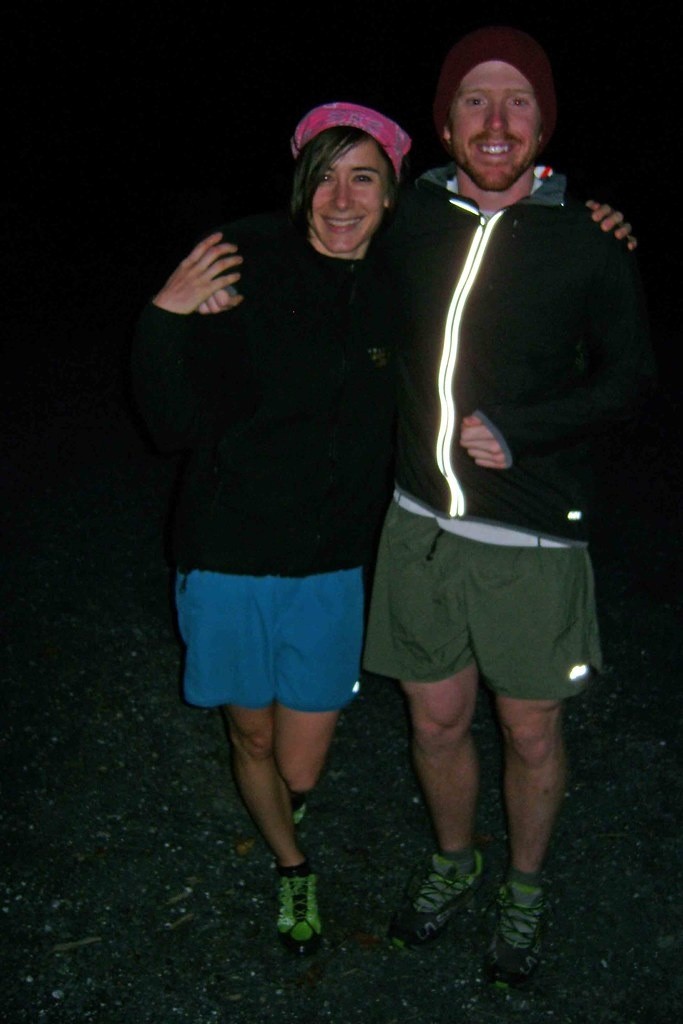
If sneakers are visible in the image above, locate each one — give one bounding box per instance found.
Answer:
[275,850,321,954]
[289,789,307,827]
[481,862,553,993]
[386,849,484,953]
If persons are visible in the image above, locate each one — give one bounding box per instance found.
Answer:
[191,26,634,990]
[133,101,638,951]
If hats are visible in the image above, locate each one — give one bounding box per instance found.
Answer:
[433,26,556,154]
[291,102,412,183]
